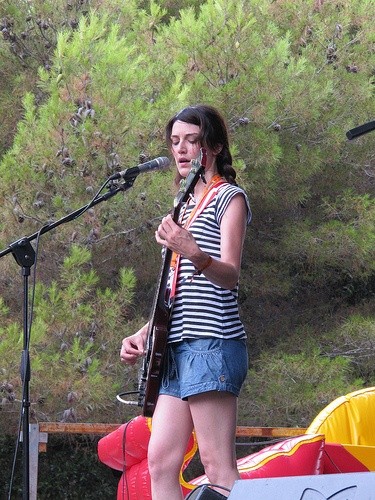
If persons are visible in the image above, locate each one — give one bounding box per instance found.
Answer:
[116,104,253,500]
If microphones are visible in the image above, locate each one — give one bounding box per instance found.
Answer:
[109,157,170,180]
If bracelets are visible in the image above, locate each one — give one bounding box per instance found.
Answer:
[194,252,212,276]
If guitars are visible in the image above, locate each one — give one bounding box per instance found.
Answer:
[138,148,207,417]
